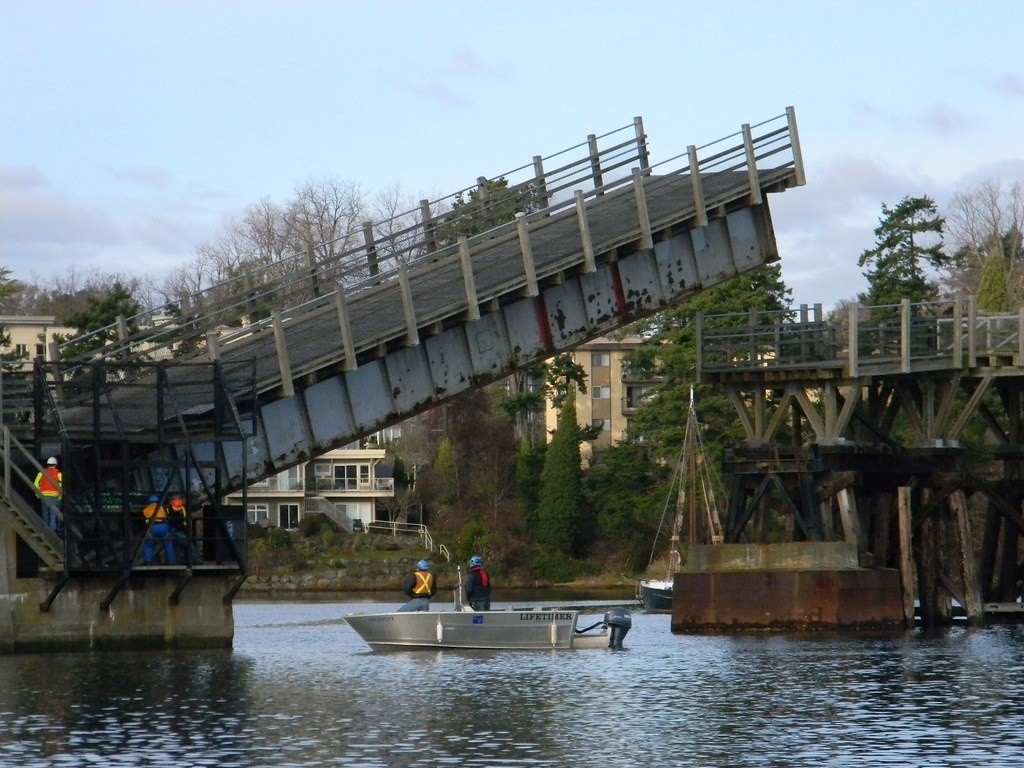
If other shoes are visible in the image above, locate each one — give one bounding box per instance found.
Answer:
[194,560,205,565]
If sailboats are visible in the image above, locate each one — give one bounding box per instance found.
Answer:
[638,386,725,613]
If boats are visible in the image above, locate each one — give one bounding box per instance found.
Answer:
[341,607,631,650]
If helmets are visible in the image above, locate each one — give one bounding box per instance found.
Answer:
[172,500,183,510]
[46,457,57,465]
[469,557,482,565]
[148,496,159,503]
[417,560,429,569]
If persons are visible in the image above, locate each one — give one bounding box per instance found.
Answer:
[143,492,178,566]
[167,499,204,566]
[34,457,62,532]
[395,560,437,613]
[464,556,490,612]
